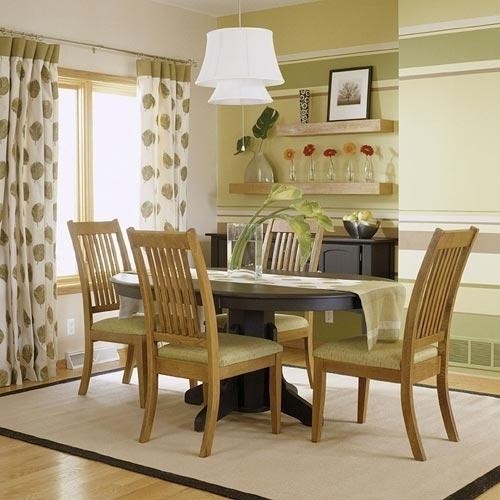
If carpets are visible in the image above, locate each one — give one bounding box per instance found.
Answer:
[0,362,499,500]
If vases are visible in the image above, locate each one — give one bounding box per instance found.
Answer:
[287,155,373,183]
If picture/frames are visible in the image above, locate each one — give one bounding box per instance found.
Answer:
[326,66,372,121]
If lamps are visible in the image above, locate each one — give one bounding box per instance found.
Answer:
[195,0,286,151]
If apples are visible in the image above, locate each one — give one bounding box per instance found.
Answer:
[342,210,378,225]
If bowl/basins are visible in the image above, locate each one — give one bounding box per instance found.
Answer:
[343,219,381,239]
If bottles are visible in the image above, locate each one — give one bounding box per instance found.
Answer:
[244,151,375,183]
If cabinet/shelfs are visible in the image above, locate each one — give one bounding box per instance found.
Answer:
[228,117,396,196]
[205,232,398,279]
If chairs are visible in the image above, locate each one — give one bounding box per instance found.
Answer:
[204,218,324,388]
[66,218,196,408]
[125,226,284,458]
[312,224,481,460]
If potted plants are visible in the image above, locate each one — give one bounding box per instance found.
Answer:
[233,107,279,183]
[225,183,334,278]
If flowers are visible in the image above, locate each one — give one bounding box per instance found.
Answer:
[282,143,375,180]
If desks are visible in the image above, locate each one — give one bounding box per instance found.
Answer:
[111,267,400,432]
